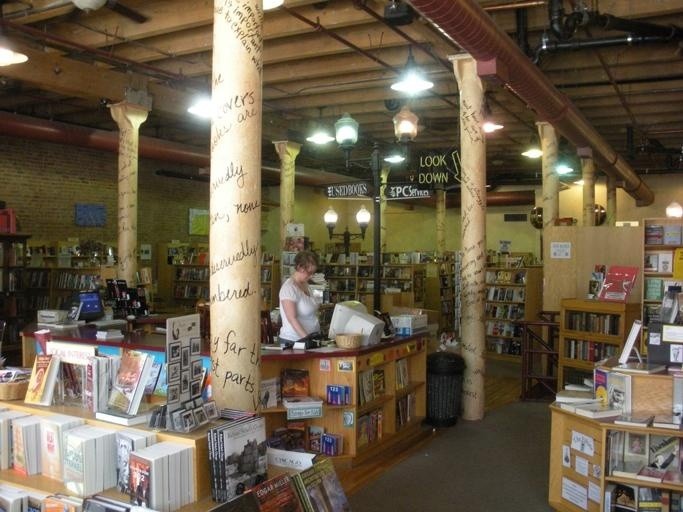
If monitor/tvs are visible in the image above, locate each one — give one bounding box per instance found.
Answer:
[328,299,386,346]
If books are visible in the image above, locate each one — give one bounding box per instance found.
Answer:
[2,223,527,511]
[552,222,682,512]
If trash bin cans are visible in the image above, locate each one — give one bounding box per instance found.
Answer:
[426,352,466,428]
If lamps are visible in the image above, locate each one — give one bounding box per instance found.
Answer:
[305,45,543,162]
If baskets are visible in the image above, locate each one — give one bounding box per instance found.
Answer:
[331,327,364,349]
